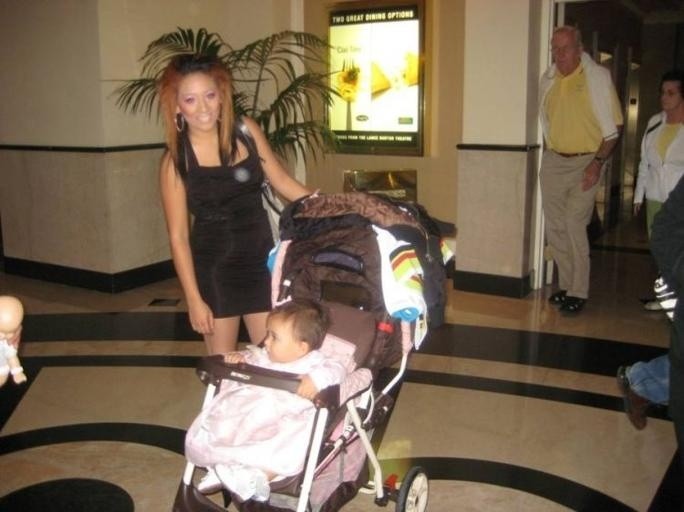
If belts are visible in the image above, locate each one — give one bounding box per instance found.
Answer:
[551,148,594,158]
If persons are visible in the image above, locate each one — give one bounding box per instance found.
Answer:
[158,55,315,356]
[633,69,683,311]
[539,25,624,316]
[617,353,671,430]
[648,174,683,512]
[184,297,346,503]
[7,328,21,349]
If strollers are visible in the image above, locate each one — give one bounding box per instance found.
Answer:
[168,192,446,510]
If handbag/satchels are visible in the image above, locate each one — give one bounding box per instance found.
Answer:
[260,179,285,248]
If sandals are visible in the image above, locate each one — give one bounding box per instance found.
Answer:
[562,297,585,313]
[548,290,567,304]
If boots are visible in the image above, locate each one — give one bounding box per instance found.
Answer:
[637,279,657,301]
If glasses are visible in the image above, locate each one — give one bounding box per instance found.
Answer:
[551,45,581,53]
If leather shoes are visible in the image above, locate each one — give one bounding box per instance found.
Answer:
[617,365,652,431]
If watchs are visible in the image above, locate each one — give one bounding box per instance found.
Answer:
[594,155,605,165]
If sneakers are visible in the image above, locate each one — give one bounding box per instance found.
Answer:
[214,463,257,503]
[643,299,663,311]
[197,469,223,495]
